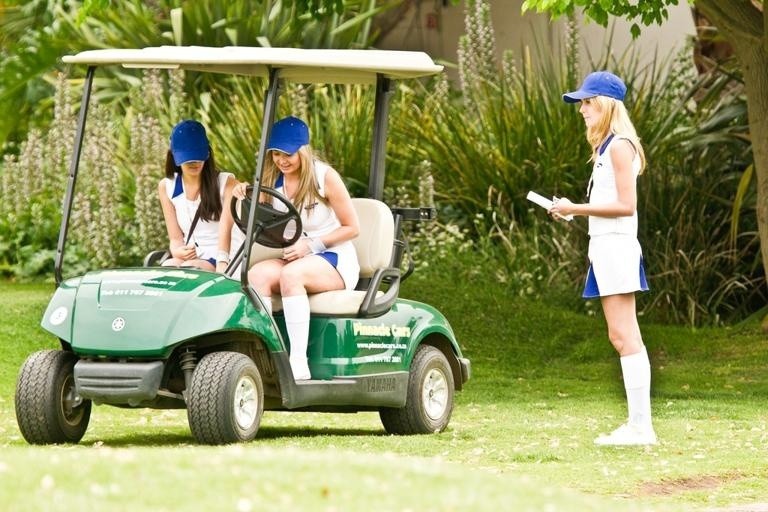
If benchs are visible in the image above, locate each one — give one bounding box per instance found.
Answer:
[143,198,403,319]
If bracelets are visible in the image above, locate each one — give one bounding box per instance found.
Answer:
[306,238,327,254]
[216,251,229,264]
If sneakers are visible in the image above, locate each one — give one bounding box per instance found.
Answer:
[598,425,655,445]
[279,353,311,383]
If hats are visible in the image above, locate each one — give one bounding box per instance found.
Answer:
[266,117,310,155]
[561,70,628,104]
[170,120,211,166]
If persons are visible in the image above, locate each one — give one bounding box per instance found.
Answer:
[224,115,363,377]
[155,118,244,281]
[547,70,660,446]
